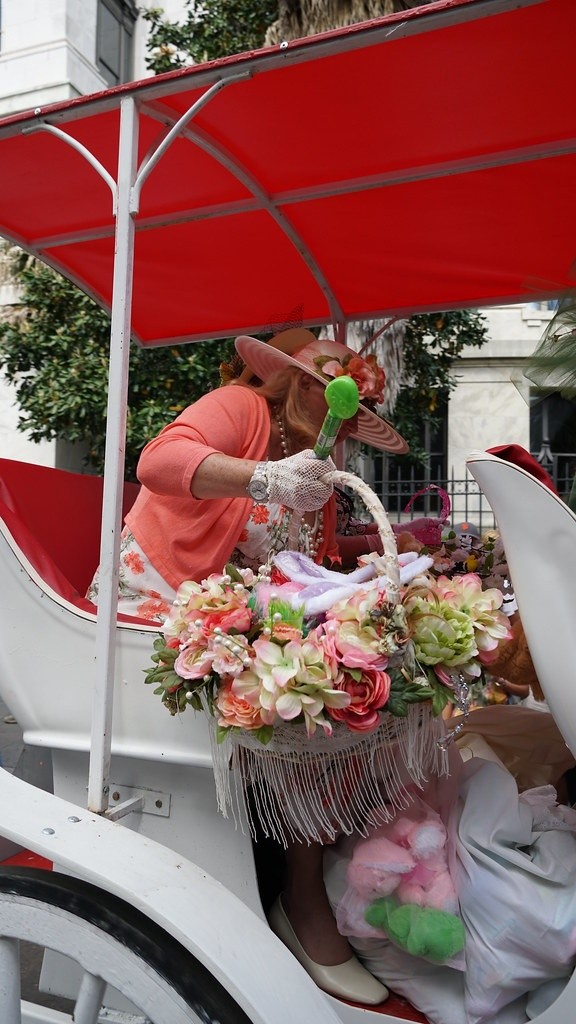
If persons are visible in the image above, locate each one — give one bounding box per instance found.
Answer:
[81,337,452,1010]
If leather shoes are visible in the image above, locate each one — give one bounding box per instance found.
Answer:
[268,891,390,1005]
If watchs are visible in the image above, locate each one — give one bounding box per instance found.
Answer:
[245,461,270,505]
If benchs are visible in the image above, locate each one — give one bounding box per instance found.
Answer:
[0,456,241,770]
[465,444,576,760]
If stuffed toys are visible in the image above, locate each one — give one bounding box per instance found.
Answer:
[336,816,464,963]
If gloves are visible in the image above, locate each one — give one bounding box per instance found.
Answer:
[365,530,425,553]
[392,518,450,545]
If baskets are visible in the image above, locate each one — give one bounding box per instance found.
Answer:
[216,469,435,761]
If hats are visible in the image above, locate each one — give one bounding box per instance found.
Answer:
[235,335,410,455]
[454,522,480,540]
[219,304,317,388]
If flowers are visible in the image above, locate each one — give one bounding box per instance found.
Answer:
[313,351,386,406]
[146,527,515,744]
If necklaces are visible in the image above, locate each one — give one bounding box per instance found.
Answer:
[273,404,323,562]
